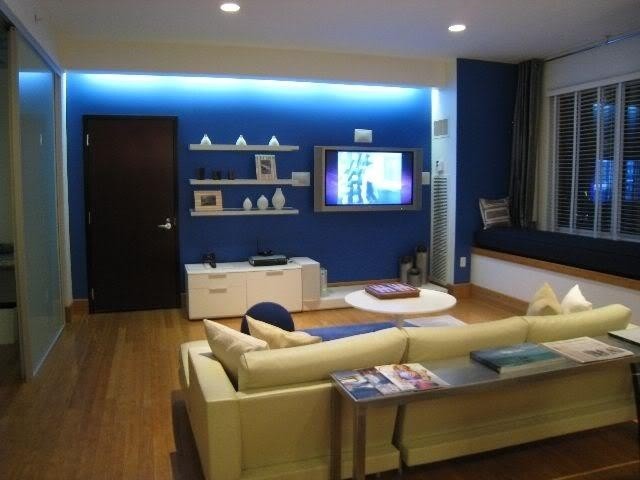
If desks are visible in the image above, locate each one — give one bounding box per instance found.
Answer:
[327,333,640,479]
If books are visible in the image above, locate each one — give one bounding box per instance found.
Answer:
[542,337,633,363]
[469,343,567,376]
[374,363,451,392]
[329,368,382,401]
[611,324,640,344]
[356,368,402,397]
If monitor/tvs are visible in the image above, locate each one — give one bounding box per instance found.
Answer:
[312,144,422,213]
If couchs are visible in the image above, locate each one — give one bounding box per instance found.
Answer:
[179,303,640,479]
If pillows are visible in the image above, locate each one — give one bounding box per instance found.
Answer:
[200,319,271,394]
[526,284,559,317]
[478,196,513,230]
[246,315,322,349]
[560,284,593,315]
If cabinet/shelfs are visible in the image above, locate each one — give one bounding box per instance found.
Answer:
[184,261,303,320]
[188,145,301,217]
